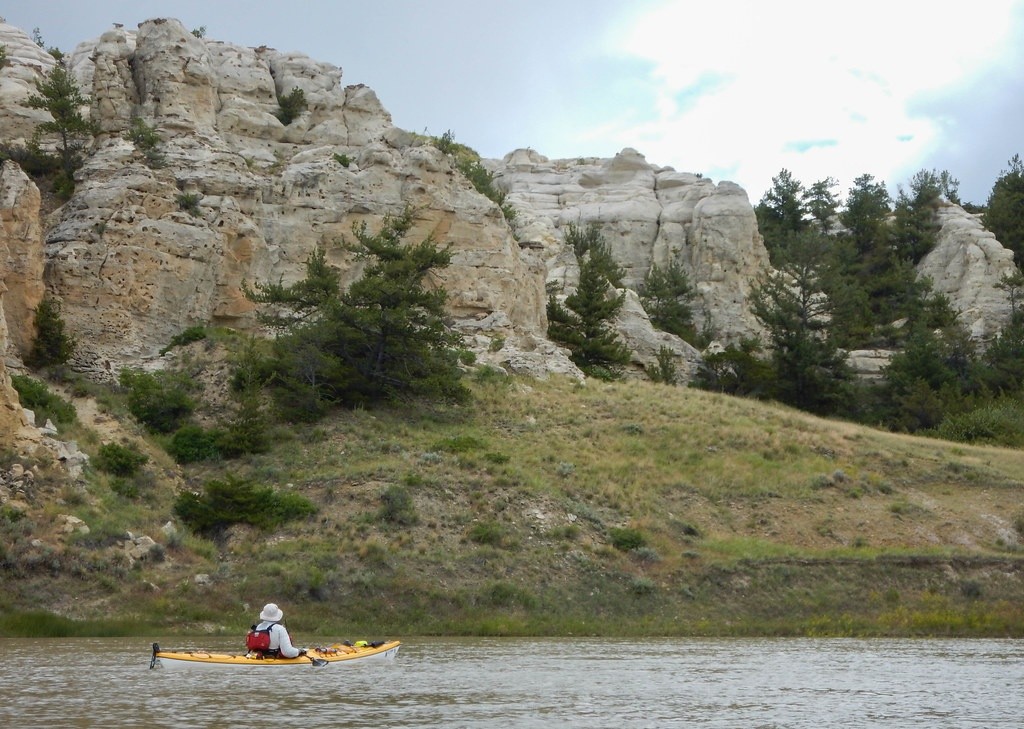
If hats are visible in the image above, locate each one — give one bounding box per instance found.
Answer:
[260,603,284,622]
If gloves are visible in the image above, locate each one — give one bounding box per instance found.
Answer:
[298,649,307,657]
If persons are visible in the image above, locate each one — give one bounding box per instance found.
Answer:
[255,603,309,659]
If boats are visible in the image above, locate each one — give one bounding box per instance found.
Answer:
[149,640,403,669]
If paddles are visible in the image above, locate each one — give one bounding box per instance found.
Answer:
[251,624,330,668]
